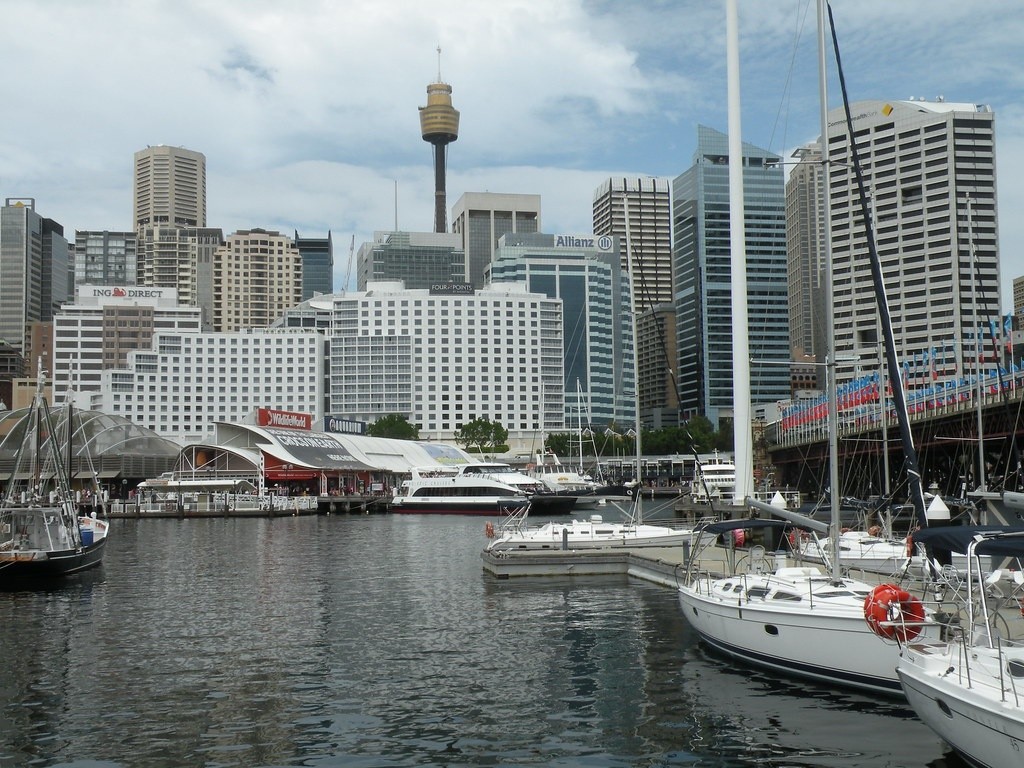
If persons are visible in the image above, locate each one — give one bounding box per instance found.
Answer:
[277,481,388,497]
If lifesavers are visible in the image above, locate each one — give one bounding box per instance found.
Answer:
[485,521,494,536]
[863,587,923,640]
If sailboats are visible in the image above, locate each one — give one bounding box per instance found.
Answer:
[484,194,721,550]
[1,351,110,573]
[676,0,950,698]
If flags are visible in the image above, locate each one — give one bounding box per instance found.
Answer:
[913,353,918,379]
[989,319,999,360]
[848,398,898,425]
[782,370,878,431]
[931,348,938,380]
[908,373,984,414]
[922,351,928,380]
[978,324,985,365]
[951,334,958,372]
[967,334,973,368]
[1004,312,1013,354]
[987,358,1024,394]
[904,358,909,390]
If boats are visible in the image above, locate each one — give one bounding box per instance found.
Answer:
[791,255,988,584]
[892,525,1024,768]
[391,461,535,517]
[698,448,735,496]
[526,451,590,494]
